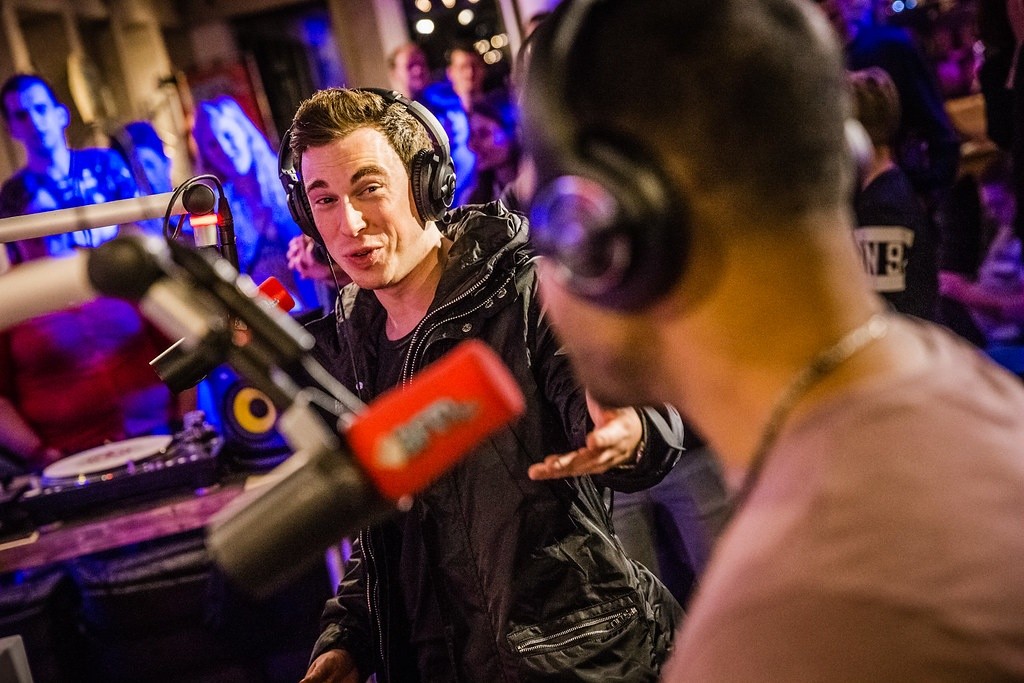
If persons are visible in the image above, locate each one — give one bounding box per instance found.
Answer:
[290,87,683,683]
[1,73,212,464]
[514,0,1024,682]
[386,13,548,207]
[849,0,1024,349]
[194,96,332,319]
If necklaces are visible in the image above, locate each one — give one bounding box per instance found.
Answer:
[739,315,888,502]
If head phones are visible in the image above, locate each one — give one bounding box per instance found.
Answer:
[522,0,876,314]
[278,87,456,249]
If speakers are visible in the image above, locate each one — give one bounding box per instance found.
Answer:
[208,362,294,475]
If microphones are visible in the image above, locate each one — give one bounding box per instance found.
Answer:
[91,197,527,598]
